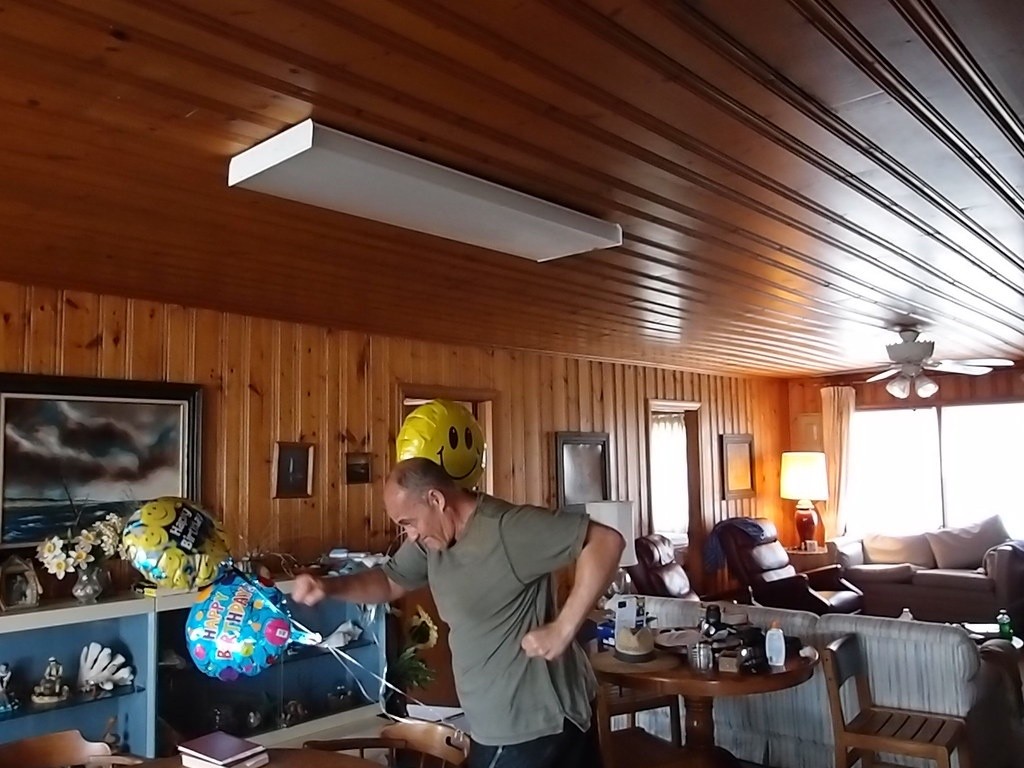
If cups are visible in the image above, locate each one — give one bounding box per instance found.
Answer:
[807,540,818,552]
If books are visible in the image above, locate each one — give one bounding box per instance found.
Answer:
[176,730,270,768]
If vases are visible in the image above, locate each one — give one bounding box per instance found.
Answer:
[72,563,102,603]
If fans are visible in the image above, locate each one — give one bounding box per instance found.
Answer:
[866,329,1014,399]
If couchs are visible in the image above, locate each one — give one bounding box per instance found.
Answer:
[827,515,1024,625]
[606,593,1024,768]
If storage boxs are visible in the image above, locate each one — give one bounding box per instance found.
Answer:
[718,650,740,673]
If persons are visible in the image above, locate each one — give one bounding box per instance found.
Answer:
[291,457,627,768]
[44,657,63,693]
[0,663,19,710]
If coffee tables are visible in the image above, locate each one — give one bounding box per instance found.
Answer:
[783,545,830,573]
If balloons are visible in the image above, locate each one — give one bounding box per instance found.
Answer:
[396,398,484,491]
[122,496,233,590]
[185,574,322,684]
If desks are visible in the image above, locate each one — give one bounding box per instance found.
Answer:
[589,643,815,768]
[125,748,386,768]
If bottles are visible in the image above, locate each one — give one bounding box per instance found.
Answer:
[765,621,786,666]
[899,608,913,620]
[691,644,712,672]
[996,609,1011,640]
[705,604,720,625]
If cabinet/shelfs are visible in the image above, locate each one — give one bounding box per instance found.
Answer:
[0,566,389,768]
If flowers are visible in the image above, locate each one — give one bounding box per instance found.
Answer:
[36,511,127,581]
[384,603,439,701]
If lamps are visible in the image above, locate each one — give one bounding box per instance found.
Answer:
[885,362,939,399]
[227,116,623,263]
[778,452,829,549]
[585,501,638,599]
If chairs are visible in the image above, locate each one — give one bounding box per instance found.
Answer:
[627,534,704,604]
[0,729,145,768]
[302,722,470,768]
[580,610,682,768]
[819,631,971,768]
[714,515,866,618]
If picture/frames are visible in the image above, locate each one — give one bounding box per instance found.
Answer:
[0,371,205,568]
[271,441,316,500]
[344,452,373,485]
[555,430,610,514]
[722,433,758,502]
